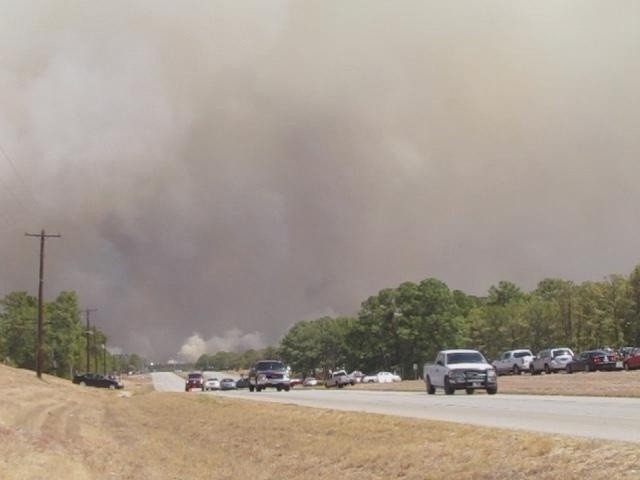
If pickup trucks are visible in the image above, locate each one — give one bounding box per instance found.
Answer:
[420,346,500,398]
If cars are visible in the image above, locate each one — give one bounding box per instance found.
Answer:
[490,344,640,376]
[72,369,125,391]
[201,368,404,390]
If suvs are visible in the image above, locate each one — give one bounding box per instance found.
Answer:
[247,358,294,393]
[184,373,205,392]
